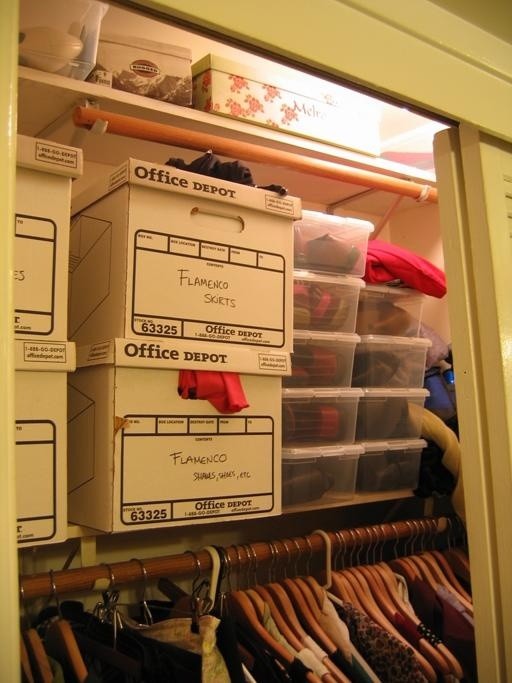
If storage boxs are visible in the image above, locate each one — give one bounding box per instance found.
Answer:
[68,333,293,536]
[357,284,426,338]
[193,51,382,158]
[360,436,427,492]
[55,156,298,353]
[281,330,360,389]
[282,387,362,448]
[356,387,431,442]
[281,442,365,510]
[351,337,429,389]
[12,340,76,550]
[290,202,375,279]
[289,270,367,335]
[72,26,194,107]
[13,132,80,342]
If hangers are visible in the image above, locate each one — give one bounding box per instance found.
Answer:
[18,510,475,682]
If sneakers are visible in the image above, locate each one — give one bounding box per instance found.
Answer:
[282,229,420,505]
